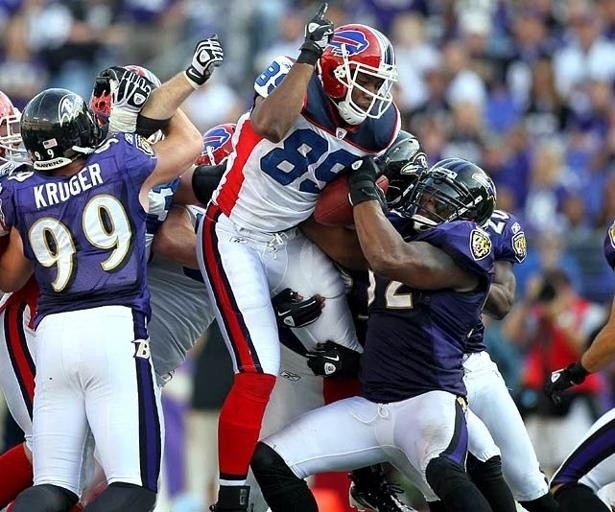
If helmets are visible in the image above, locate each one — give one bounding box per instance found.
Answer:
[313,24,399,126]
[90,66,164,143]
[379,130,427,208]
[0,90,32,177]
[195,123,235,164]
[405,157,496,232]
[20,89,102,170]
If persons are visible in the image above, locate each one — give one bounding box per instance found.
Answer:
[1,0,614,512]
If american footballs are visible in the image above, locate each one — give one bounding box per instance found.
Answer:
[314,178,389,227]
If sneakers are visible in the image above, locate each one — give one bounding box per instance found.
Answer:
[349,475,419,512]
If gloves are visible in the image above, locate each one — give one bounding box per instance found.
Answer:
[297,2,334,64]
[184,34,224,86]
[346,155,389,203]
[272,288,324,328]
[307,342,361,384]
[543,360,592,406]
